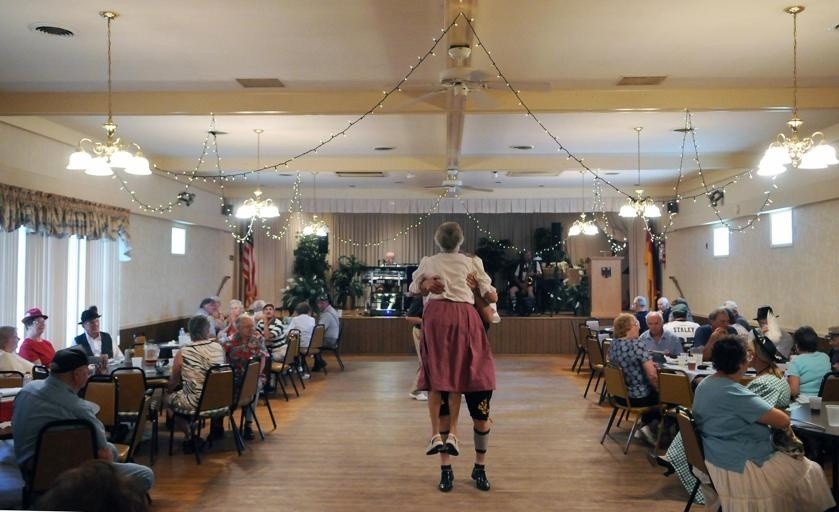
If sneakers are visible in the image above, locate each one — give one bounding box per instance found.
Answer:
[244,425,255,439]
[312,360,327,371]
[408,390,429,401]
[289,367,303,373]
[634,425,656,446]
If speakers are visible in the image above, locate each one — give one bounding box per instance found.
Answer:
[222,204,233,214]
[667,202,679,213]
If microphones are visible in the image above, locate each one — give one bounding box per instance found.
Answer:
[599,249,610,254]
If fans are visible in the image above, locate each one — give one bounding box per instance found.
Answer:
[394,43,554,108]
[421,165,494,200]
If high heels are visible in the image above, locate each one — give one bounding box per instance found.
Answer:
[646,450,676,477]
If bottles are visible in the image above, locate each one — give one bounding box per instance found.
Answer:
[178,326,187,350]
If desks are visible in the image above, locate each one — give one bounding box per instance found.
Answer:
[539,273,588,317]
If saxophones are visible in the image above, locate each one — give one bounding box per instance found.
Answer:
[521,259,533,295]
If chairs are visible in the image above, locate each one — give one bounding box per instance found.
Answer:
[571,313,839,511]
[509,264,542,318]
[148,309,346,464]
[0,310,161,511]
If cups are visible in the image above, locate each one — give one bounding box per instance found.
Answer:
[676,351,704,373]
[131,356,143,372]
[88,363,96,376]
[808,395,823,410]
[101,353,109,367]
[144,343,162,362]
[168,357,175,373]
[824,403,839,429]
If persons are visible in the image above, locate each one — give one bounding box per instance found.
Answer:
[1,295,125,389]
[364,253,399,316]
[607,297,837,399]
[189,296,340,442]
[652,337,791,507]
[478,238,543,316]
[408,224,498,457]
[406,296,430,401]
[691,336,835,511]
[144,317,227,445]
[409,276,501,492]
[13,347,154,508]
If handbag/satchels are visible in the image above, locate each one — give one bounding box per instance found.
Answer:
[770,426,806,458]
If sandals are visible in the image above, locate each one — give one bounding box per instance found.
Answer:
[445,433,459,456]
[426,433,443,456]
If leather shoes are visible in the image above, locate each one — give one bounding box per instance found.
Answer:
[472,467,491,491]
[439,470,455,491]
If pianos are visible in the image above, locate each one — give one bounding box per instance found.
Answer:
[359,265,418,291]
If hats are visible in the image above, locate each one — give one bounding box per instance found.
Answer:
[753,327,787,363]
[78,310,101,325]
[825,326,839,338]
[21,308,48,321]
[50,349,100,372]
[672,303,688,313]
[753,306,779,321]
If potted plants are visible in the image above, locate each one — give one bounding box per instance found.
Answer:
[329,253,371,311]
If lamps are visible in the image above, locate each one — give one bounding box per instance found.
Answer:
[567,173,600,238]
[756,4,839,177]
[301,172,330,238]
[234,128,280,220]
[617,126,663,219]
[65,10,153,177]
